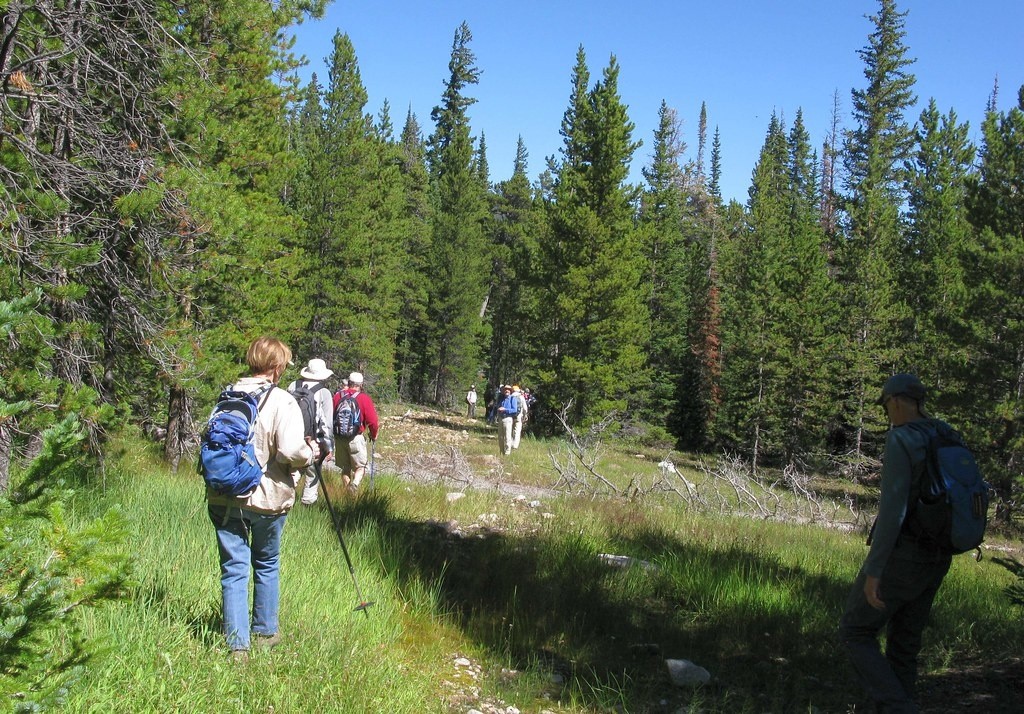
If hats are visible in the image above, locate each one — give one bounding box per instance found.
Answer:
[349,372,363,384]
[300,358,334,380]
[874,374,925,405]
[503,385,514,395]
[512,386,519,390]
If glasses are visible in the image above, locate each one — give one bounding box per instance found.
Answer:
[881,395,899,413]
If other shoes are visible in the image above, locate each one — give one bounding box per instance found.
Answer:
[225,650,249,661]
[349,483,357,493]
[256,633,280,646]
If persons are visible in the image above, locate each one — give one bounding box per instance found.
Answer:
[467,385,477,419]
[332,371,378,500]
[839,372,971,714]
[335,379,349,394]
[204,335,321,663]
[286,358,334,505]
[484,381,536,456]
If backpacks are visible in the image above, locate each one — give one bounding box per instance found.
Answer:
[333,390,361,437]
[197,382,275,499]
[511,396,521,417]
[291,380,323,441]
[894,421,989,555]
